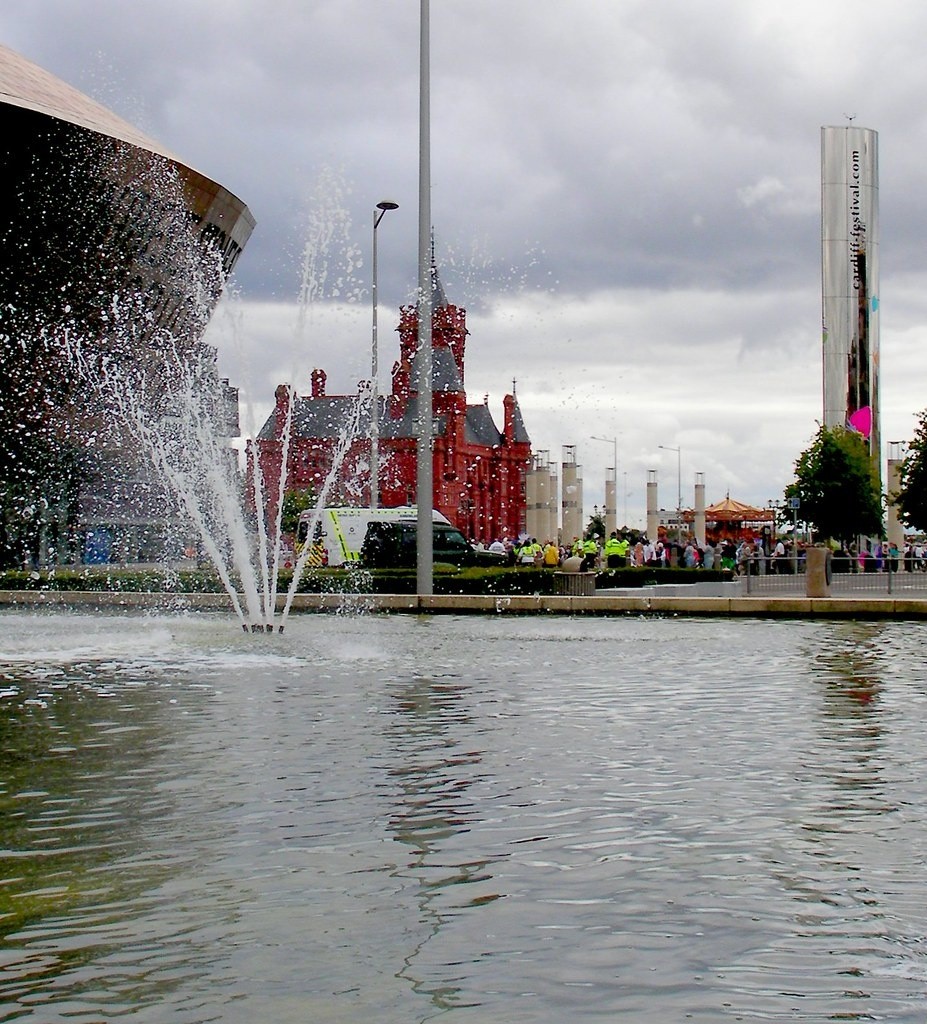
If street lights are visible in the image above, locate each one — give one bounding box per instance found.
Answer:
[659,445,681,544]
[372,198,399,509]
[594,504,606,537]
[768,499,780,539]
[591,436,617,481]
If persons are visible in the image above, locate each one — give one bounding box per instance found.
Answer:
[478,532,927,575]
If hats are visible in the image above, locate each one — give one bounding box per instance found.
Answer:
[587,534,592,540]
[610,532,616,538]
[719,540,728,545]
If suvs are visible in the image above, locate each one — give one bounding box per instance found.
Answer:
[360,521,509,568]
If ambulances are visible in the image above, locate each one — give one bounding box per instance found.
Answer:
[293,504,478,568]
